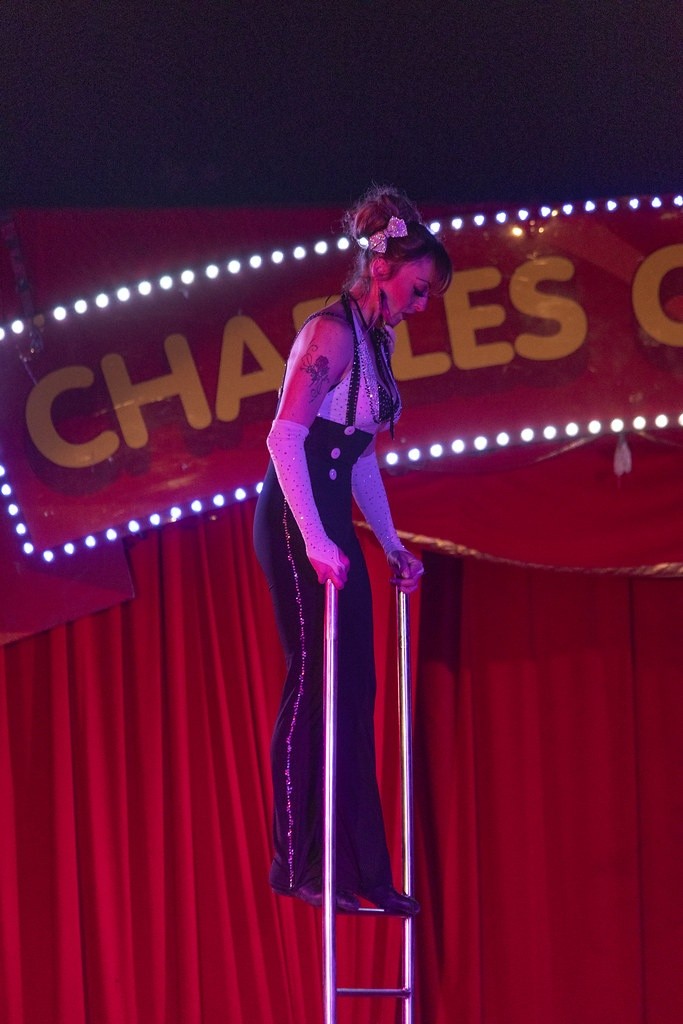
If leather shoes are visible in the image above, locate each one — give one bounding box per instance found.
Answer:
[272,874,420,918]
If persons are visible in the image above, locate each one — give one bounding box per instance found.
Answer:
[252,188,453,918]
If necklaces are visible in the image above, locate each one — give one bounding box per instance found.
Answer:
[350,295,394,440]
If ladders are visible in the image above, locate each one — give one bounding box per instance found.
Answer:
[321,559,414,1024]
